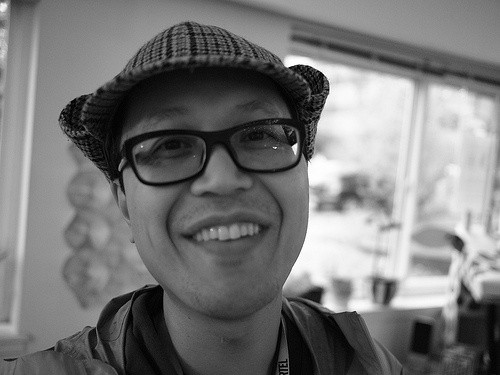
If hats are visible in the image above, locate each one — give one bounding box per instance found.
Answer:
[58,21,329,182]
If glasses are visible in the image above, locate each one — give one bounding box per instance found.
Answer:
[119,117,307,187]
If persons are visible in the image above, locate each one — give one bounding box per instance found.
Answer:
[1,19,423,374]
